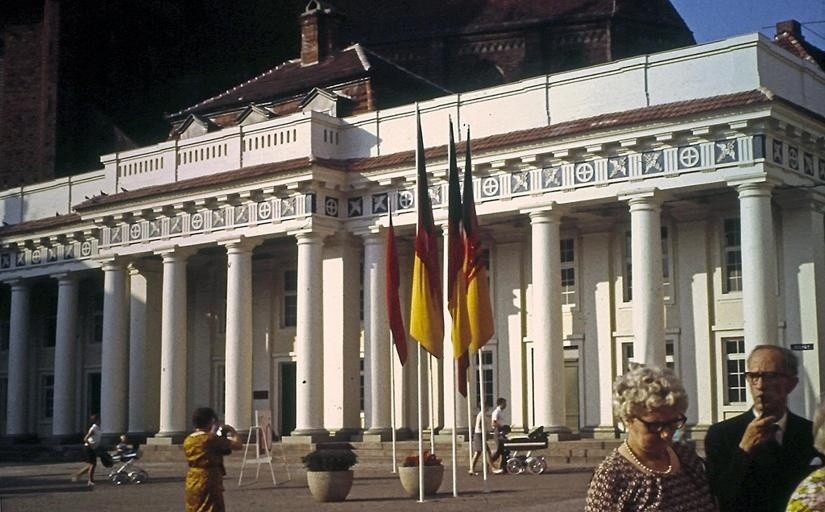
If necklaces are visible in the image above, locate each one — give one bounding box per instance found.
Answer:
[624,439,672,475]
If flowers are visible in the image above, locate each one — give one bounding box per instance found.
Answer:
[403,451,443,466]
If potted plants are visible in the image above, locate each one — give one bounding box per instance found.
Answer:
[300,449,359,502]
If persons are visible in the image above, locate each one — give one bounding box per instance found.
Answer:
[491,397,506,469]
[72,415,101,486]
[704,346,825,512]
[583,366,719,512]
[784,400,824,512]
[673,424,689,456]
[183,407,243,512]
[468,398,505,475]
[117,435,130,454]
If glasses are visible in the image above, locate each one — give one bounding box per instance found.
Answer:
[634,413,687,433]
[745,371,790,382]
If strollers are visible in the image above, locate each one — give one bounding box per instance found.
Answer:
[95,433,148,485]
[500,424,548,474]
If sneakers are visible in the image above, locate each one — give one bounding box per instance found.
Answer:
[493,469,503,475]
[469,470,478,475]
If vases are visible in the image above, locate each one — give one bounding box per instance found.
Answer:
[398,464,444,496]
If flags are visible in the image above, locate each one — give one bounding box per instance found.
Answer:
[459,128,495,354]
[409,109,443,358]
[387,226,407,365]
[448,124,470,396]
